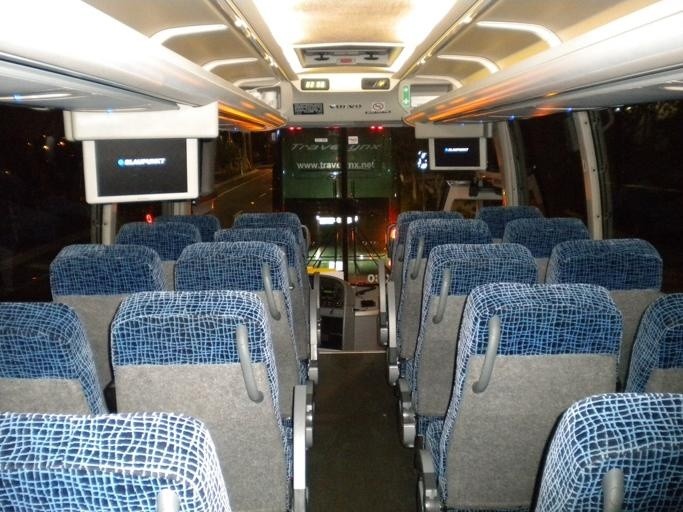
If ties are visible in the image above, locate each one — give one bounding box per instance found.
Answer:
[428,137,488,171]
[82,138,199,205]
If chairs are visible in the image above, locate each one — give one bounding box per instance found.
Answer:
[378,204,683,512]
[0,212,321,512]
